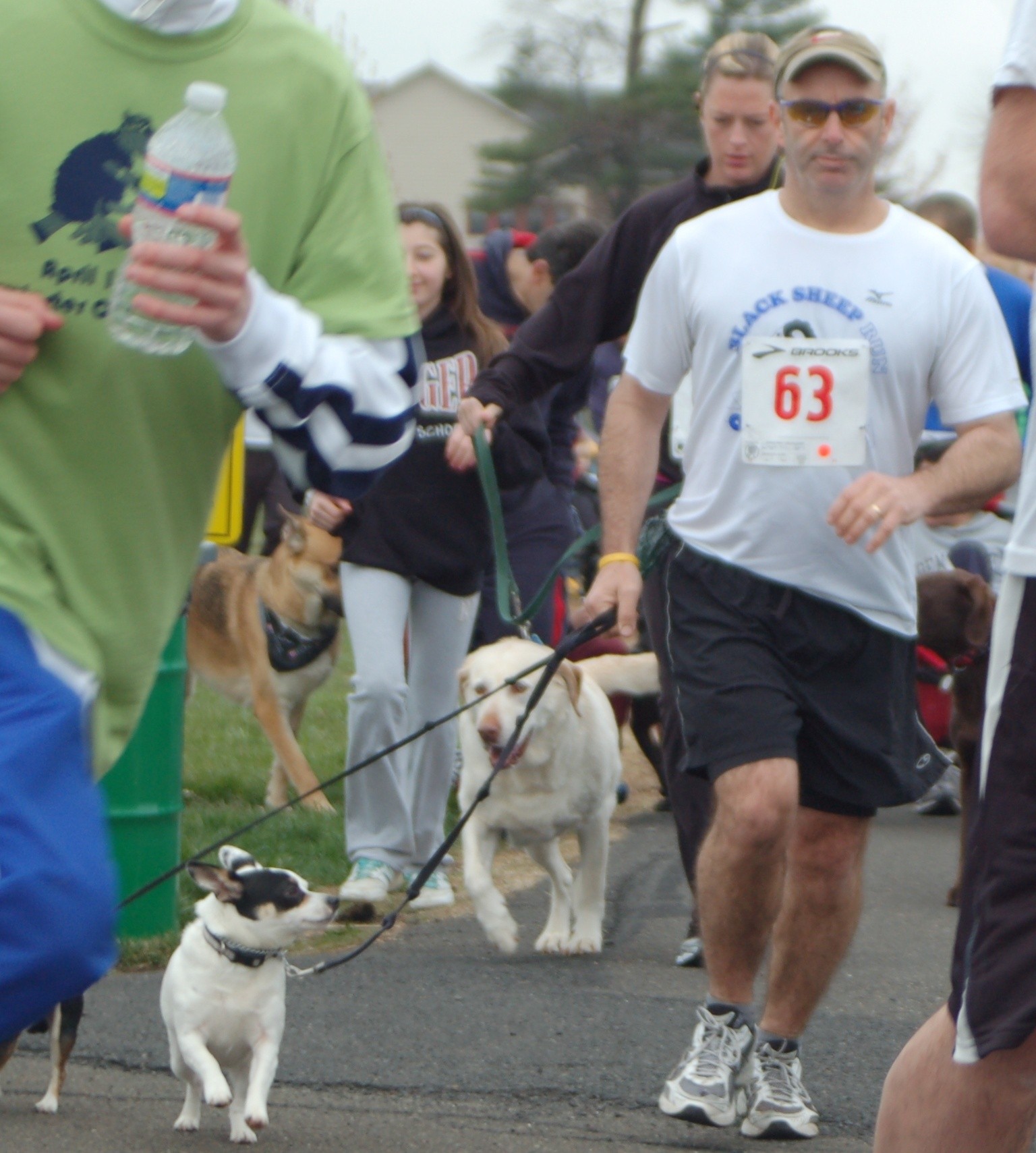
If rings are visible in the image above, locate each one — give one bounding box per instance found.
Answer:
[872,504,884,515]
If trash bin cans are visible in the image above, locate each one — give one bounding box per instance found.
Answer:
[97,602,188,940]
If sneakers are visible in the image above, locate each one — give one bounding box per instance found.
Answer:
[404,866,455,911]
[659,1001,761,1125]
[739,1039,821,1138]
[338,855,398,904]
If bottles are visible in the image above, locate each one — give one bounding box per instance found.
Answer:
[102,77,241,357]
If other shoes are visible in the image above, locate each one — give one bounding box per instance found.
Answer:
[913,761,963,815]
[676,934,705,968]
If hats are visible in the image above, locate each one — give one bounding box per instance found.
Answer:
[773,25,888,108]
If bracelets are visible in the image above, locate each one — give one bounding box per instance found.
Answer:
[595,553,641,568]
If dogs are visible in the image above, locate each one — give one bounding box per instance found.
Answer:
[1,992,85,1111]
[457,637,659,955]
[186,497,346,816]
[156,841,340,1142]
[912,568,1000,746]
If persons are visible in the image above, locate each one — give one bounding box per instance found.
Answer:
[0,0,425,1066]
[232,0,1035,1153]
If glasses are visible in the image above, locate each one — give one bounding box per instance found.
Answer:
[781,97,883,128]
